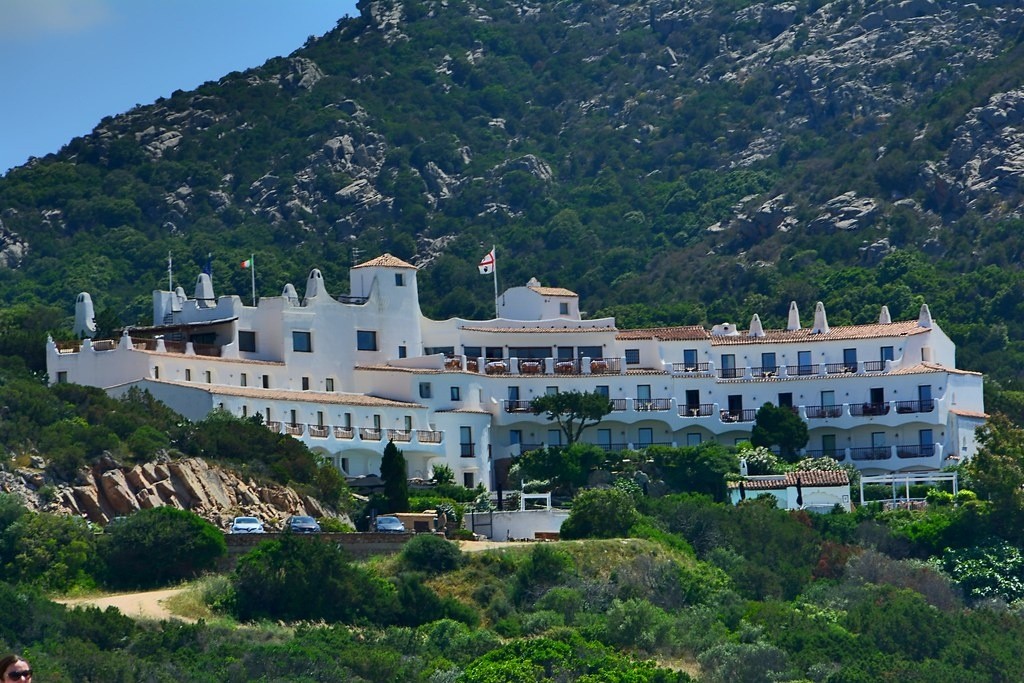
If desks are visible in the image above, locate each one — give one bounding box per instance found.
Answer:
[644,402,655,411]
[521,362,542,374]
[486,362,506,374]
[686,367,695,372]
[589,360,607,374]
[556,362,573,373]
[443,359,460,367]
[688,409,700,417]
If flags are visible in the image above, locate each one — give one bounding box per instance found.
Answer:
[478,249,494,275]
[203,258,210,278]
[241,259,252,269]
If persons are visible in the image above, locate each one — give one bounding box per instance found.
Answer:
[0,654,33,683]
[438,508,447,531]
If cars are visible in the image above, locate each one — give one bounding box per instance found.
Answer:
[103,515,130,534]
[281,515,322,533]
[369,515,405,533]
[228,517,266,533]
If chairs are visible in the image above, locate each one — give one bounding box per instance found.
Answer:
[679,366,685,371]
[638,403,643,411]
[697,366,703,372]
[655,403,660,410]
